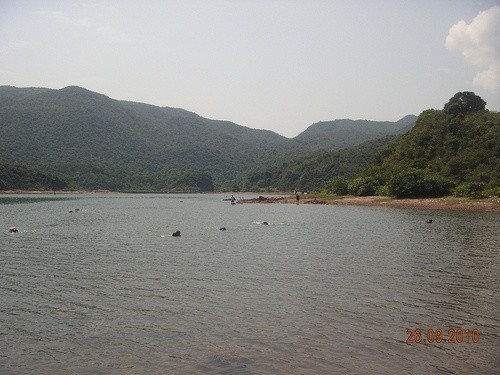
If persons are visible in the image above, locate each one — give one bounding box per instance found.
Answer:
[296,194,300,204]
[231,195,235,205]
